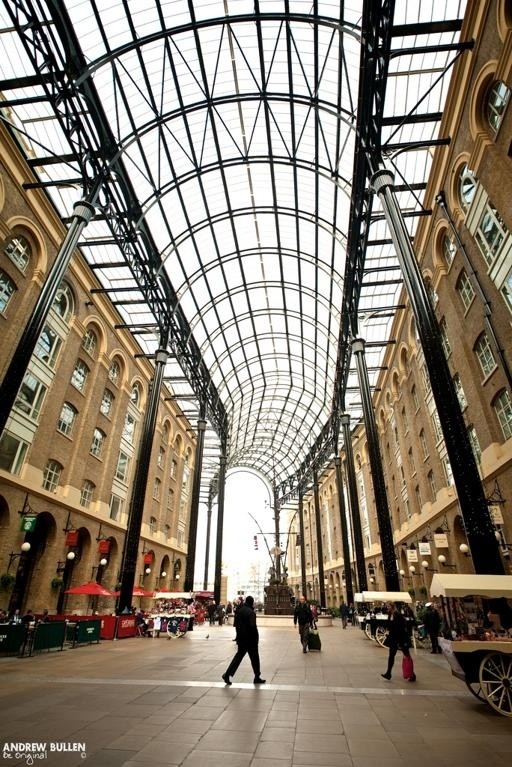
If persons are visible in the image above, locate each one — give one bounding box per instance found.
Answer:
[291,594,314,654]
[0,607,52,629]
[336,599,512,657]
[222,595,267,685]
[381,601,417,683]
[124,597,243,638]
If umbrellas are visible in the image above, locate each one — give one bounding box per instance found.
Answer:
[64,581,121,616]
[111,586,155,600]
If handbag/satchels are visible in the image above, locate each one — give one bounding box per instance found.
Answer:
[402,656,414,681]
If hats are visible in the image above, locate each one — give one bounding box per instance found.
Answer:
[425,602,432,607]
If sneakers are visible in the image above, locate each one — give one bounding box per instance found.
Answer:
[429,649,442,655]
[381,672,392,680]
[221,672,234,685]
[253,678,266,684]
[408,673,417,681]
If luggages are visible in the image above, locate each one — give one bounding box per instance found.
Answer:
[307,629,322,652]
